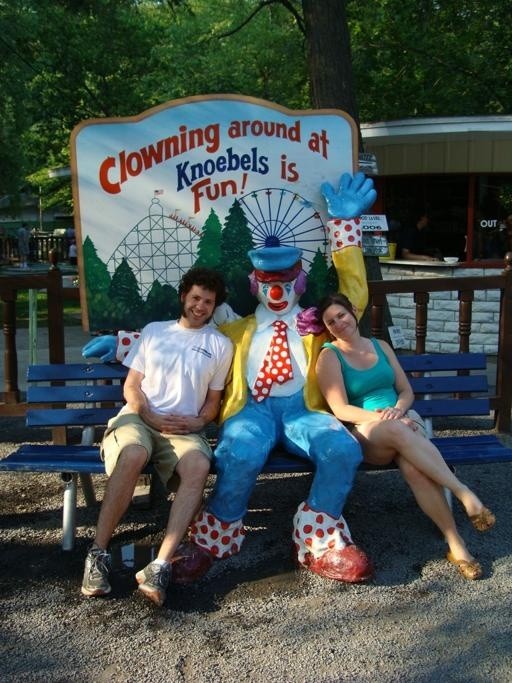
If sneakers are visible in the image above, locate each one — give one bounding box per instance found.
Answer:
[134,562,169,608]
[80,548,112,597]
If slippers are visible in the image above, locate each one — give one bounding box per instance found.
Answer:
[447,549,483,579]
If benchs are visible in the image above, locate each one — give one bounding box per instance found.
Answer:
[0,352,511,552]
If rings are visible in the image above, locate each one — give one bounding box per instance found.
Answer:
[390,410,396,414]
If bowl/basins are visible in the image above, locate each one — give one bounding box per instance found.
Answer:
[444,257,459,263]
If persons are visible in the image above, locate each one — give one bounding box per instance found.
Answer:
[401,208,444,263]
[17,222,32,270]
[494,213,512,260]
[78,266,236,605]
[82,171,374,582]
[63,222,78,272]
[312,289,500,580]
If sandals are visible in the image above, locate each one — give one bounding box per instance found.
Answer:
[463,504,497,533]
